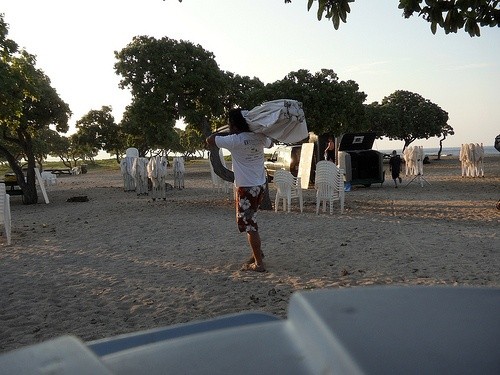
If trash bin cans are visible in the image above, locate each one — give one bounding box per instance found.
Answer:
[4,173,24,195]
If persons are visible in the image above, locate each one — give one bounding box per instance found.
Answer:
[207,107,273,271]
[323,138,336,166]
[389,150,404,187]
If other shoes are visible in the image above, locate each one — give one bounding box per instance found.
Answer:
[241,262,265,272]
[399,177,402,182]
[248,251,265,263]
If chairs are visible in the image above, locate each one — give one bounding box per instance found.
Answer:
[271,170,304,213]
[315,161,344,214]
[0,183,12,245]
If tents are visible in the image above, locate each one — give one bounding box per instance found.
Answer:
[459,142,485,178]
[120,154,186,201]
[215,100,308,144]
[403,146,423,176]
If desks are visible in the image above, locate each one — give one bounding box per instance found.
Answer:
[43,168,71,177]
